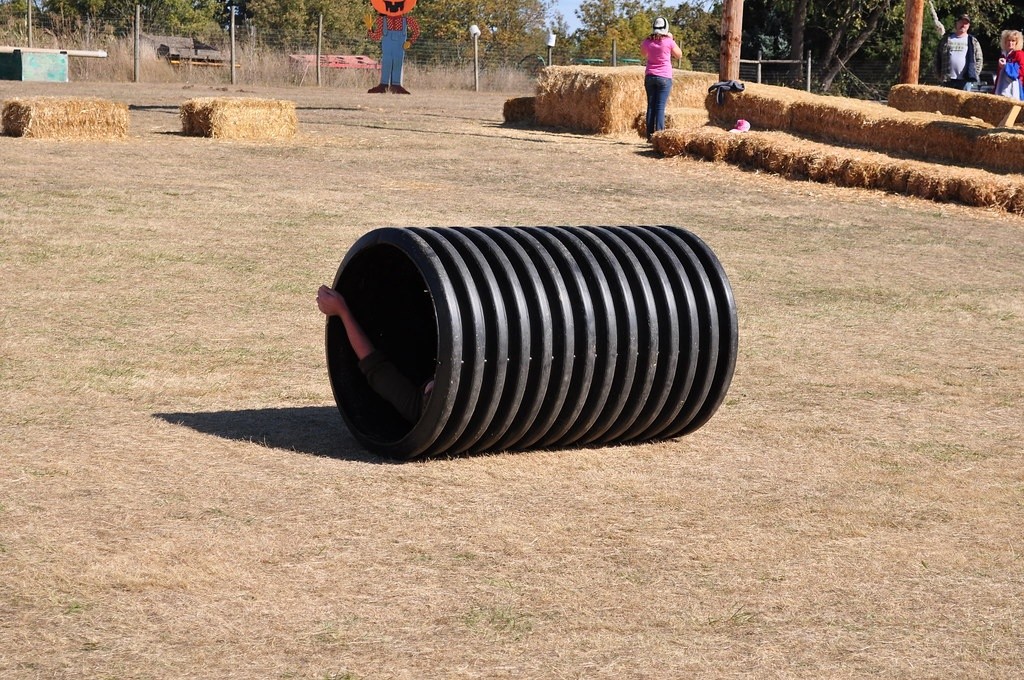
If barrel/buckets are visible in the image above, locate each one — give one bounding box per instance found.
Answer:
[325,224,739,463]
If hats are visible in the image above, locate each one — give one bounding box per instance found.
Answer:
[959,14,971,22]
[652,16,669,35]
[729,119,750,134]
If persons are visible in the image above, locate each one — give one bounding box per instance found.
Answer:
[936,14,983,92]
[363,0,419,95]
[992,30,1024,101]
[316,285,435,425]
[641,16,682,143]
